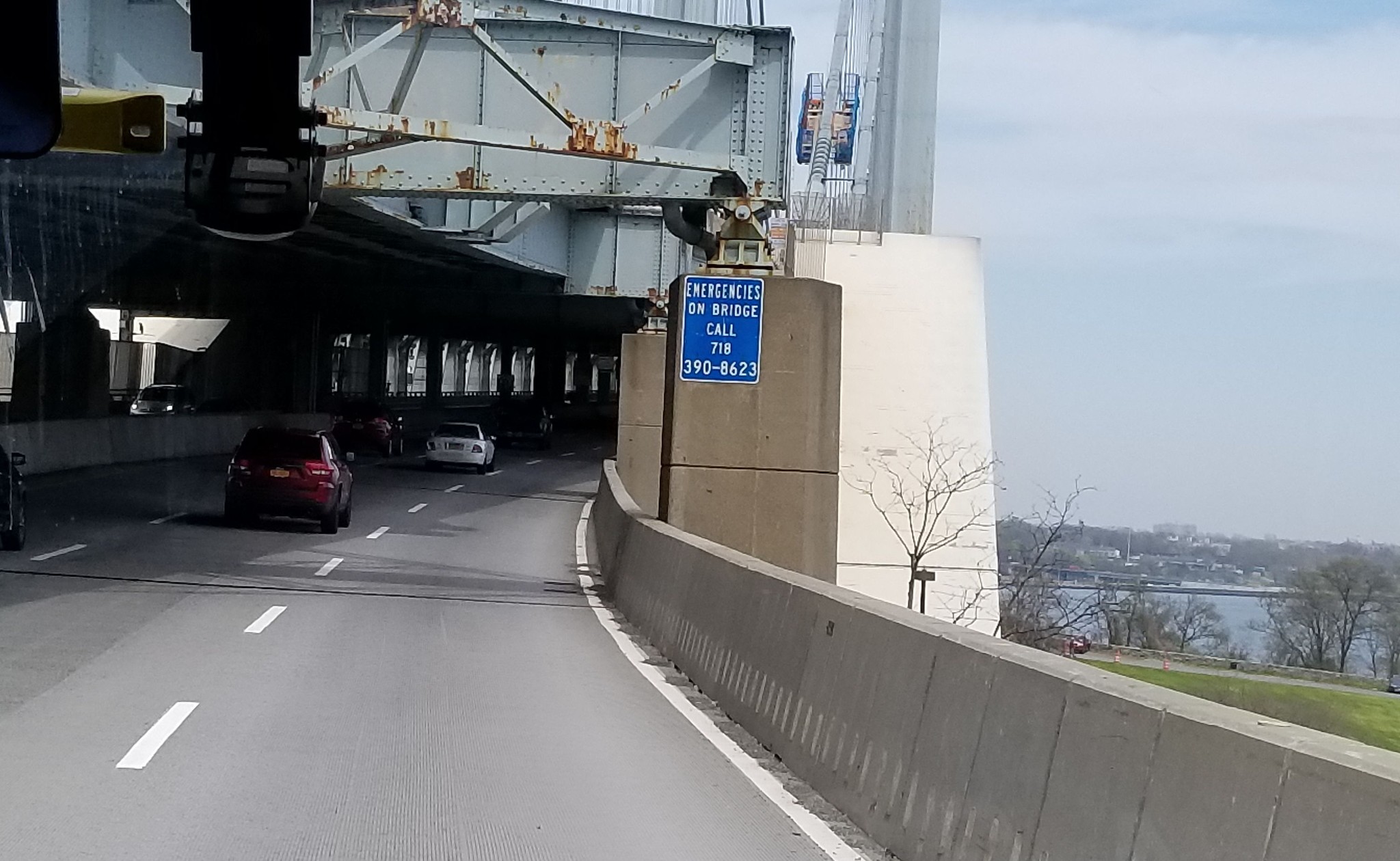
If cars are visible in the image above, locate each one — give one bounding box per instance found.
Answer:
[425,422,496,477]
[494,400,556,451]
[129,384,198,416]
[333,409,407,459]
[0,445,31,550]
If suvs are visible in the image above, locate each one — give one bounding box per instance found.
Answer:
[222,425,356,536]
